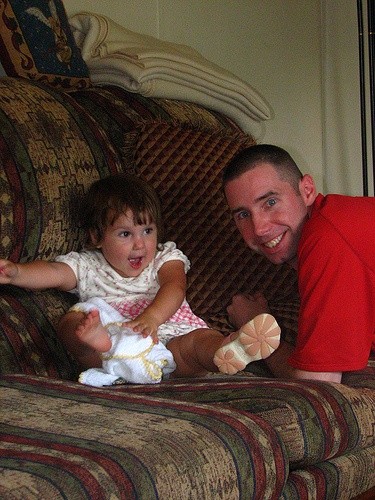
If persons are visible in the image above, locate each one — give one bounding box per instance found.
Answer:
[222,144,375,383]
[0,173,281,380]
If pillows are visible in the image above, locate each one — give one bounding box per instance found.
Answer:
[0,0,90,91]
[118,114,300,348]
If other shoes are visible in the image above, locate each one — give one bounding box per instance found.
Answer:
[213,313,281,375]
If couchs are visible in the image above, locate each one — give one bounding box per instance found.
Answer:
[0,85,375,500]
